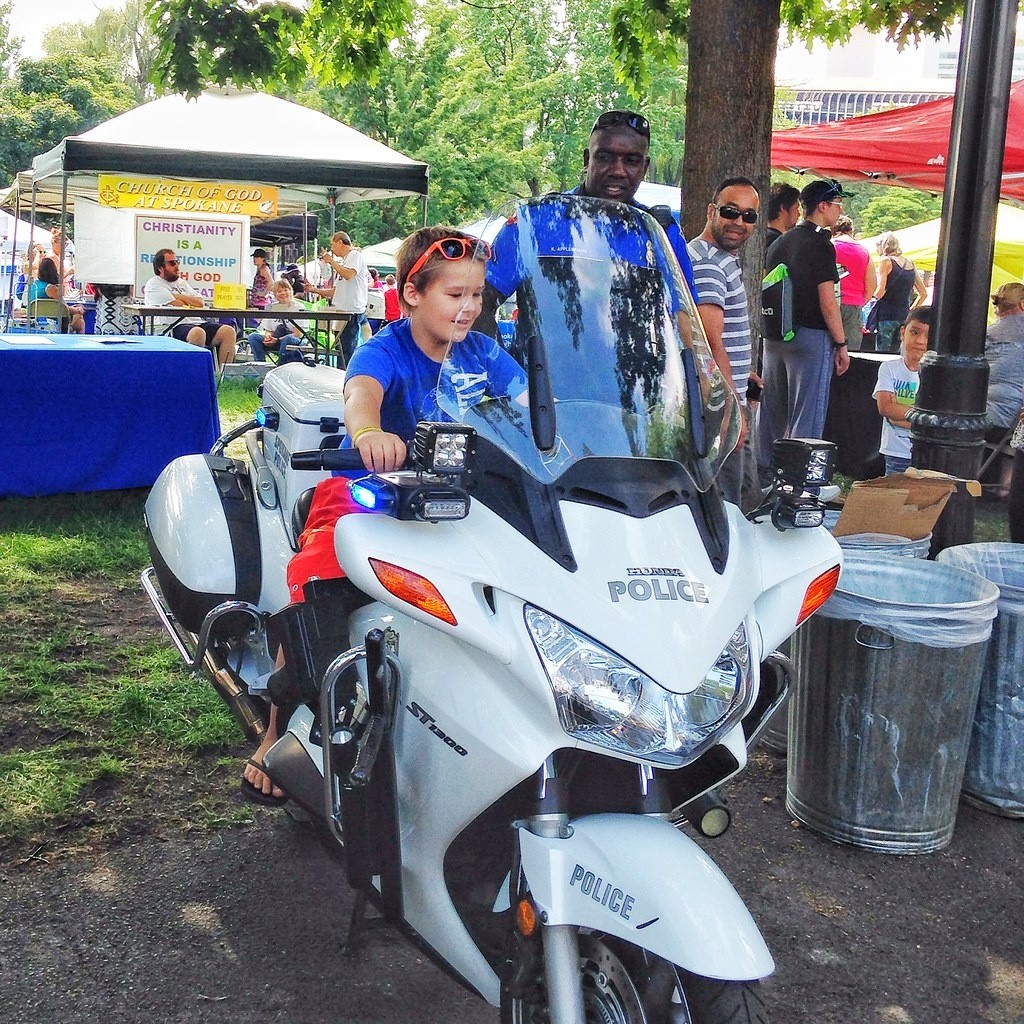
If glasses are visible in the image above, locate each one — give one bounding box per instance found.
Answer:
[163,260,179,266]
[406,238,491,282]
[713,203,758,224]
[590,111,651,150]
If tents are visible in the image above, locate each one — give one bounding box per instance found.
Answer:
[770,76,1024,206]
[0,85,430,332]
[858,203,1024,328]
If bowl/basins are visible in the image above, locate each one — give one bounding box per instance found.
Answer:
[84,295,95,301]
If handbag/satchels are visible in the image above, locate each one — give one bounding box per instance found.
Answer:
[863,297,878,332]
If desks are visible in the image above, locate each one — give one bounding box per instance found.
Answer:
[822,348,925,480]
[120,304,354,368]
[0,333,224,500]
[64,300,146,336]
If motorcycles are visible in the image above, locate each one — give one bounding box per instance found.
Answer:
[140,196,844,1024]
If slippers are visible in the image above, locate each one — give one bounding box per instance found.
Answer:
[242,760,289,807]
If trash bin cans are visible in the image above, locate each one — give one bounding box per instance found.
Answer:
[785,551,1000,854]
[821,510,933,559]
[936,541,1024,820]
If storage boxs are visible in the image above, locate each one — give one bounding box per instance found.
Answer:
[365,288,386,320]
[140,452,266,635]
[260,361,348,509]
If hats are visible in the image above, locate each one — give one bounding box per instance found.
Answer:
[798,179,857,208]
[250,248,266,257]
[286,264,301,275]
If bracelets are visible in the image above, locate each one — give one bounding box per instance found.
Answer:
[352,425,383,449]
[833,336,848,348]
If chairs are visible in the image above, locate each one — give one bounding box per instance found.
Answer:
[976,408,1024,495]
[27,299,72,334]
[141,298,351,376]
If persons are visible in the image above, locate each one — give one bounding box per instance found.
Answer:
[15,227,400,372]
[871,305,931,478]
[982,281,1024,445]
[474,111,711,454]
[327,226,560,485]
[828,214,877,353]
[763,182,802,246]
[681,177,765,515]
[873,230,928,354]
[766,178,856,504]
